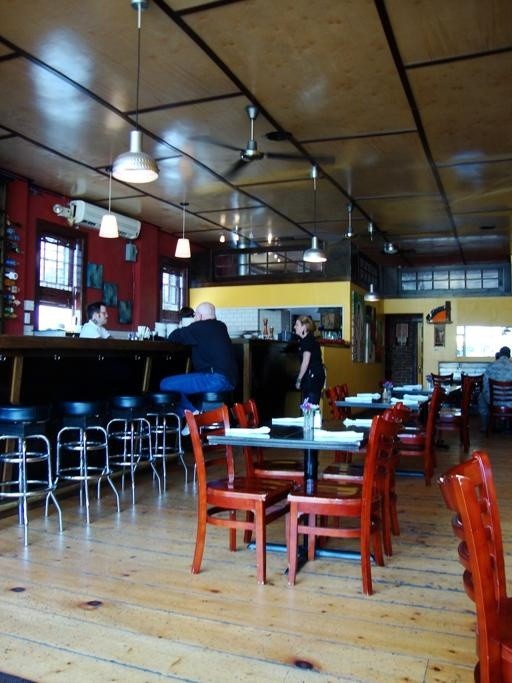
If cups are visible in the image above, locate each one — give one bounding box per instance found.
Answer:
[128,327,136,340]
[150,331,157,342]
[138,326,146,340]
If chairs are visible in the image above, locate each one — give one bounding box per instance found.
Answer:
[430,373,453,387]
[318,402,403,556]
[183,404,294,586]
[460,373,485,396]
[437,376,471,454]
[234,399,305,543]
[435,448,512,683]
[485,378,512,438]
[328,383,352,462]
[396,384,439,489]
[302,166,327,263]
[286,409,392,595]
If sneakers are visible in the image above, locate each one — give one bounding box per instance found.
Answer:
[181,423,190,435]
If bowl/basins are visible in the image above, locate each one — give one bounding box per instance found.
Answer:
[244,334,253,339]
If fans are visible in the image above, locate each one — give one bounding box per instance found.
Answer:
[186,103,335,180]
[319,204,387,247]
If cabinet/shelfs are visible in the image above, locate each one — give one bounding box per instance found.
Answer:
[0,337,249,485]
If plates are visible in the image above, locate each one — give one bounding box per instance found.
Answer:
[228,331,246,338]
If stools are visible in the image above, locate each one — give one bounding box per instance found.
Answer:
[96,395,161,505]
[134,392,188,494]
[44,399,120,524]
[187,393,226,485]
[0,404,63,548]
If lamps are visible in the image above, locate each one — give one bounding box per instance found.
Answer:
[111,1,161,184]
[363,282,380,303]
[174,202,191,258]
[98,167,119,239]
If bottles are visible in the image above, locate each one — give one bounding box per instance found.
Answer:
[3,219,25,319]
[382,388,388,400]
[314,410,322,429]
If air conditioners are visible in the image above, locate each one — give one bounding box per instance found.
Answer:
[71,200,141,241]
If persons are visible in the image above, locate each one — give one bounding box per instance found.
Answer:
[290,315,325,416]
[79,301,113,339]
[161,300,236,394]
[477,345,512,429]
